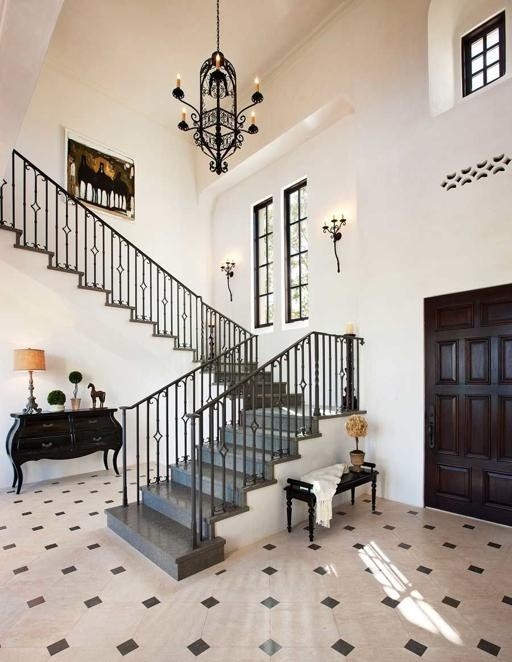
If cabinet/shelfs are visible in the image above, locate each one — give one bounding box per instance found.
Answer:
[5,406,123,494]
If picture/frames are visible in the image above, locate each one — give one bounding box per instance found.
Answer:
[64,128,136,223]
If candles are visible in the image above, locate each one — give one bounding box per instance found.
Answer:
[346,322,355,334]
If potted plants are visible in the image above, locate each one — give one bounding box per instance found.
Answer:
[69,371,83,411]
[47,390,66,413]
[345,414,368,474]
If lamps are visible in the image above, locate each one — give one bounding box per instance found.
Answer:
[319,214,349,274]
[220,261,236,302]
[172,0,264,175]
[12,348,46,415]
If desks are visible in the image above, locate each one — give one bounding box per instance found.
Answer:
[283,461,379,541]
